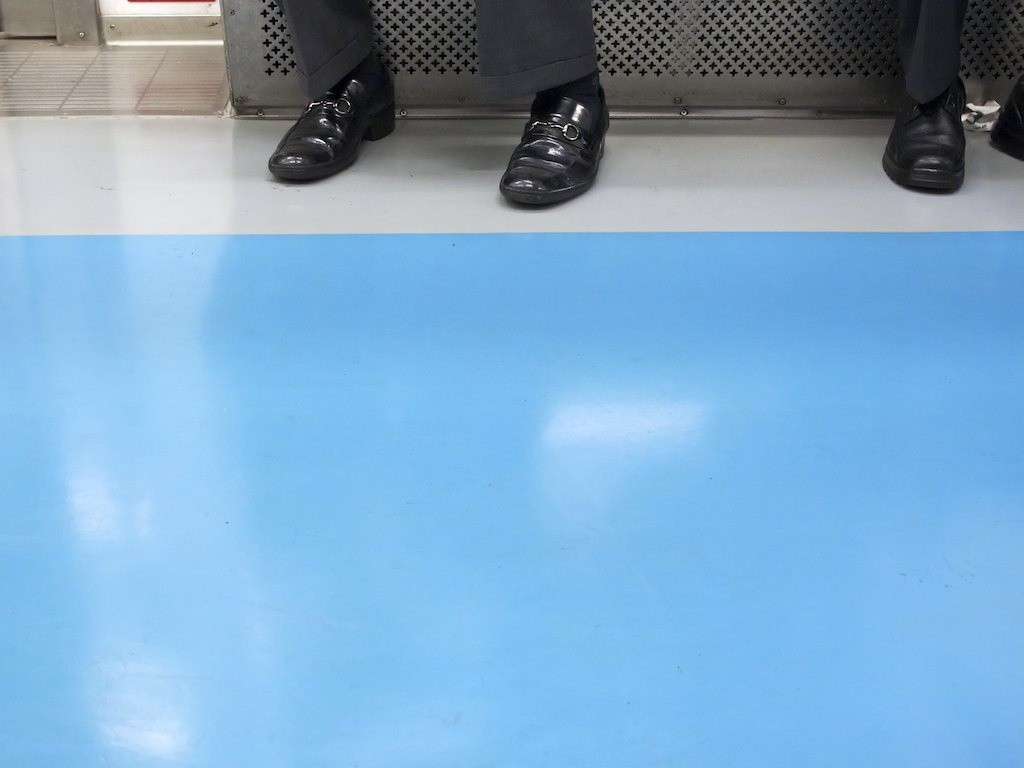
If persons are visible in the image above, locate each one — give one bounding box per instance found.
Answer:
[882,0,1024,188]
[269,0,610,203]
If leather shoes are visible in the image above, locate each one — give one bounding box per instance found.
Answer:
[883,75,966,189]
[499,84,610,204]
[990,73,1024,161]
[268,63,396,180]
